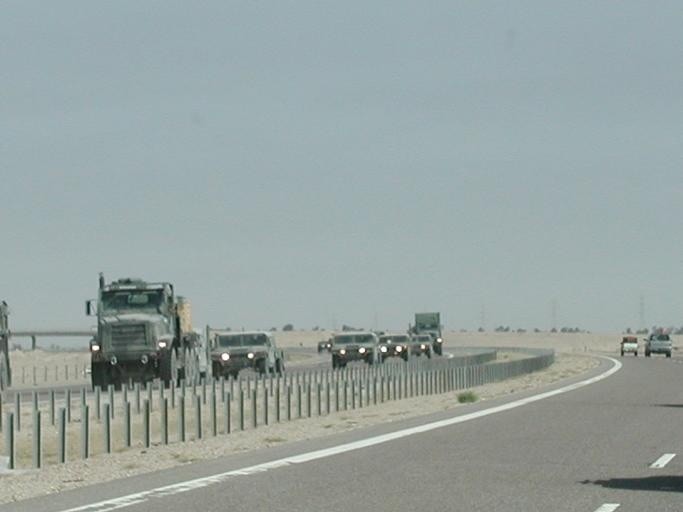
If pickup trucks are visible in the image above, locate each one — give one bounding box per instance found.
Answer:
[643,333,673,358]
[619,336,640,357]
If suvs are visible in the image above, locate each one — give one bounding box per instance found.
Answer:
[379,334,411,362]
[330,331,382,368]
[210,330,283,378]
[317,339,330,351]
[408,334,432,359]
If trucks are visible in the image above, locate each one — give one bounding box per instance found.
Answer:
[85,272,202,394]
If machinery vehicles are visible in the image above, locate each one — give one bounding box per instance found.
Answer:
[407,312,442,355]
